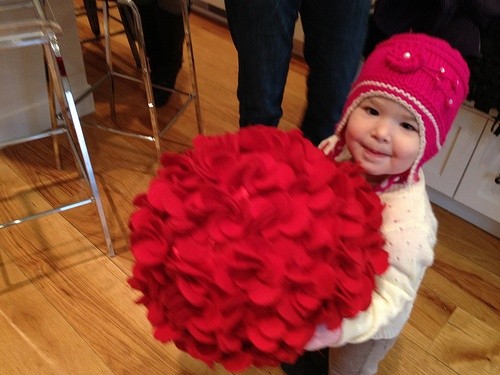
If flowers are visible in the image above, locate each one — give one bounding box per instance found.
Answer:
[126,125,389,375]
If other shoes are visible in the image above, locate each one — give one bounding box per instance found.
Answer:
[148,74,177,108]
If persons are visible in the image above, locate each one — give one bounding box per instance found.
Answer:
[225,0,370,148]
[279,33,470,375]
[128,0,192,108]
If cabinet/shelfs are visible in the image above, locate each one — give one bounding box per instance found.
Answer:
[422,100,500,239]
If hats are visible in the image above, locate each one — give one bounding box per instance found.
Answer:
[322,31,471,194]
